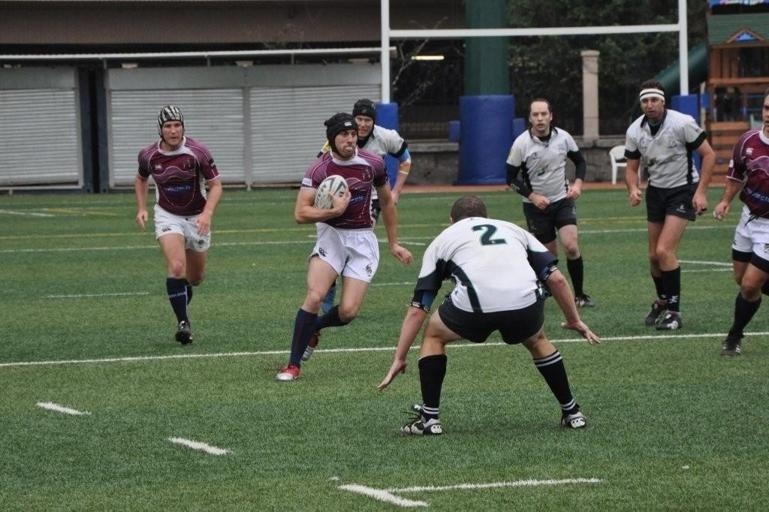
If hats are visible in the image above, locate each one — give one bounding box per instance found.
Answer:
[353,99,374,117]
[324,113,358,144]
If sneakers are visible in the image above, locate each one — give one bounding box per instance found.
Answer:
[275,364,299,381]
[560,412,586,428]
[175,321,192,344]
[400,404,442,436]
[646,300,663,326]
[721,340,741,357]
[301,333,319,361]
[575,296,595,306]
[320,287,336,313]
[656,312,681,330]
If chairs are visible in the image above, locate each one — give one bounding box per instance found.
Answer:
[608,145,642,186]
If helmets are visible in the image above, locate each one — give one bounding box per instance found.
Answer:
[158,105,184,136]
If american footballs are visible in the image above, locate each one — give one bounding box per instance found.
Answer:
[315,174,348,209]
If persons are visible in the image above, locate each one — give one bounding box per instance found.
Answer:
[316,95,413,315]
[712,85,769,359]
[133,104,224,349]
[503,96,595,311]
[376,193,600,434]
[620,78,718,333]
[274,113,414,380]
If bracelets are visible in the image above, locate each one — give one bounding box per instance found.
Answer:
[203,208,214,217]
[396,168,409,177]
